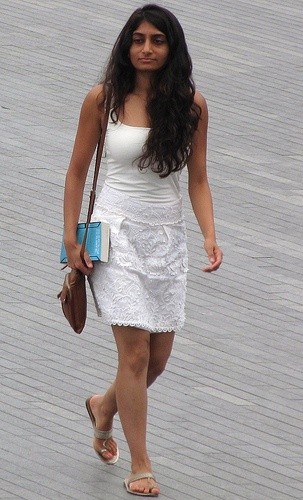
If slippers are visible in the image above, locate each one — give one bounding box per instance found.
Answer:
[85,396,120,463]
[124,473,160,496]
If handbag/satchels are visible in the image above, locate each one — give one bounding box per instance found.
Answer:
[58,269,87,333]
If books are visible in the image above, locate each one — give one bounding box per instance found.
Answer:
[59,220,111,263]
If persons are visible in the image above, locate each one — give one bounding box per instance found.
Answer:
[56,2,223,497]
